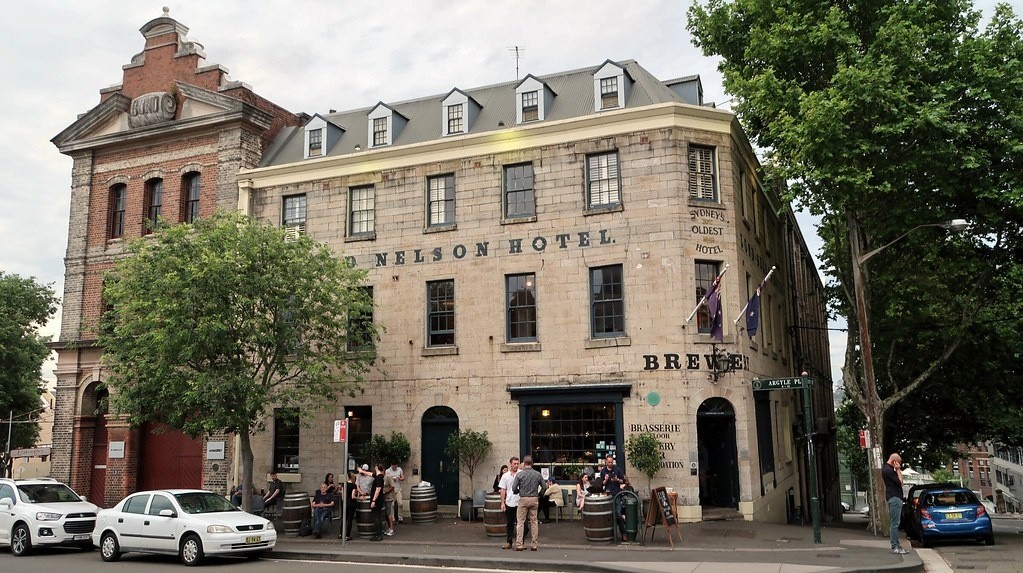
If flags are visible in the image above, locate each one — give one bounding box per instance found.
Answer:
[703,273,723,343]
[746,280,766,338]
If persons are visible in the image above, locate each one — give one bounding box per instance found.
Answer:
[311,461,405,541]
[882,453,911,554]
[232,469,285,515]
[494,455,630,551]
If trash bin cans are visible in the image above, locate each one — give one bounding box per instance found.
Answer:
[625,498,638,542]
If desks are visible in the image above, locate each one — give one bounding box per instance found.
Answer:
[468,490,487,524]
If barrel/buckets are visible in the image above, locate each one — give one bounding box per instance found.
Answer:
[583,494,614,542]
[484,492,508,536]
[356,496,387,539]
[409,485,437,524]
[282,492,311,537]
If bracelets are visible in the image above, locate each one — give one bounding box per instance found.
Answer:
[372,500,375,502]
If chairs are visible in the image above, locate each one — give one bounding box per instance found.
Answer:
[955,493,966,504]
[252,494,267,516]
[314,490,341,530]
[260,487,286,521]
[49,491,60,502]
[555,489,583,525]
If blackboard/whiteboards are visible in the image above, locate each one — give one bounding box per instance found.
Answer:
[645,487,679,530]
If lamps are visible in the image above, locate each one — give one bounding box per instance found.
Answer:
[707,343,738,385]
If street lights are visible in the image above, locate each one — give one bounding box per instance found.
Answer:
[854,219,971,537]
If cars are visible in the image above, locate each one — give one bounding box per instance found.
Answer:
[0,476,104,557]
[841,502,851,513]
[916,489,996,547]
[89,488,279,566]
[859,506,870,516]
[902,483,959,539]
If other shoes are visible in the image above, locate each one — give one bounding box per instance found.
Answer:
[531,547,537,551]
[384,529,395,536]
[370,535,384,542]
[313,529,321,540]
[622,534,628,541]
[577,508,582,515]
[513,546,524,551]
[502,543,512,549]
[545,519,549,523]
[892,547,909,554]
[338,534,352,541]
[524,547,527,549]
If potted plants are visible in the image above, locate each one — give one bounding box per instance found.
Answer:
[563,457,588,480]
[444,428,492,521]
[621,430,664,524]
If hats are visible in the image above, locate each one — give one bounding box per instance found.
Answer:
[360,464,369,471]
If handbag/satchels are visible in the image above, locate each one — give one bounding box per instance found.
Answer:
[300,519,313,536]
